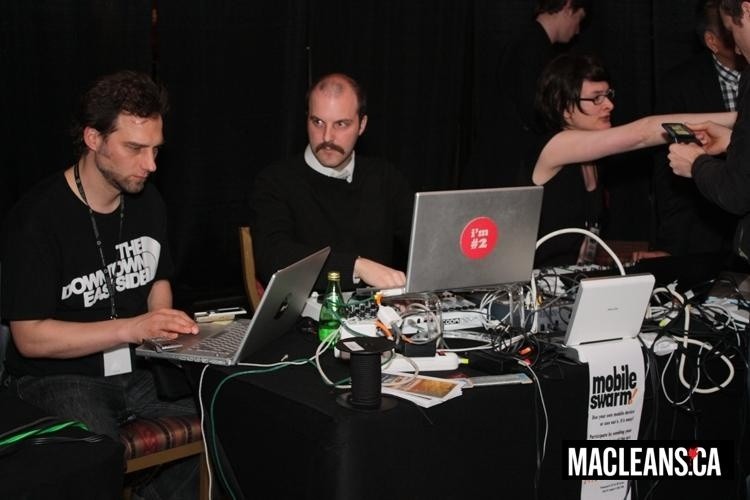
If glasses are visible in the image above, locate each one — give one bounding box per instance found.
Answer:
[568,90,615,105]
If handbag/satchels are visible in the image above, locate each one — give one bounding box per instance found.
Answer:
[0,389,127,499]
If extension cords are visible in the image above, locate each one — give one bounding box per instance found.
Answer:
[380,349,470,374]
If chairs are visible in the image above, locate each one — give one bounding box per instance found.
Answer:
[122,398,214,499]
[238,218,267,316]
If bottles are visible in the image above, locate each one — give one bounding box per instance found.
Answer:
[319,272,345,346]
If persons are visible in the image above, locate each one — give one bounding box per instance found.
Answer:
[666,1,750,261]
[0,69,200,499]
[494,2,588,151]
[253,72,417,290]
[651,1,743,167]
[507,51,738,268]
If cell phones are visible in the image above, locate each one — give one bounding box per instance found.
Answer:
[143,337,183,354]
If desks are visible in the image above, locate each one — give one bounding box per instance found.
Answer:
[193,279,750,500]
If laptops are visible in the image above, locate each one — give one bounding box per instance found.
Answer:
[357,185,547,300]
[555,273,656,364]
[135,246,332,368]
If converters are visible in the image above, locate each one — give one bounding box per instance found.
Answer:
[470,350,518,375]
[404,341,437,356]
[682,287,712,305]
